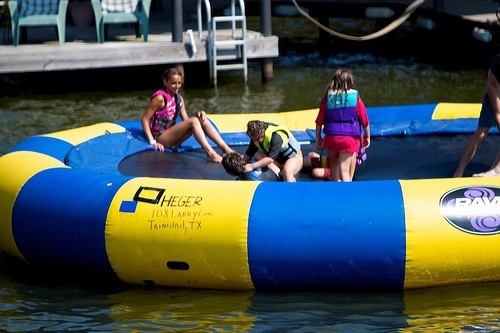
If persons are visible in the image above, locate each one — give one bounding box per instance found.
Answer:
[141,65,243,164]
[452,55,500,177]
[303,69,369,183]
[222,119,303,182]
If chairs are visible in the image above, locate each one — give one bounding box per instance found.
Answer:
[91,0,152,43]
[7,0,68,47]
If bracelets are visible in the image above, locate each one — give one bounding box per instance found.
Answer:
[150,140,157,144]
